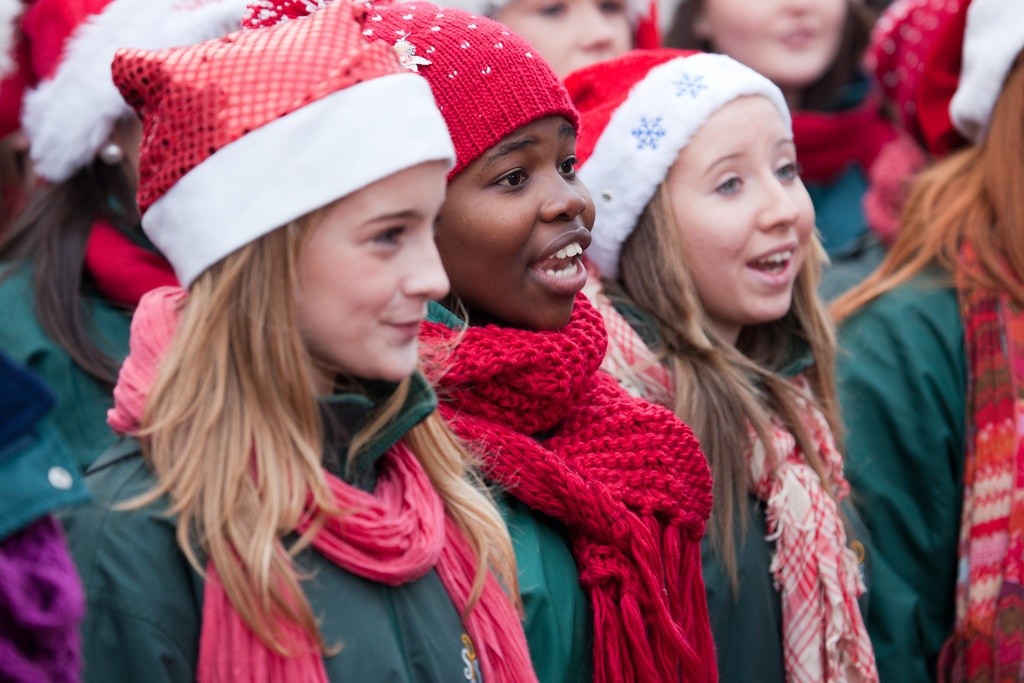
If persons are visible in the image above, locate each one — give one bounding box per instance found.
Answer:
[561,48,877,683]
[837,0,1024,683]
[0,357,94,683]
[1,0,1024,469]
[369,0,720,683]
[73,0,538,683]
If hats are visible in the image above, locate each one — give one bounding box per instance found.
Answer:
[241,1,580,183]
[0,0,248,183]
[559,46,791,283]
[860,0,1024,161]
[110,0,452,289]
